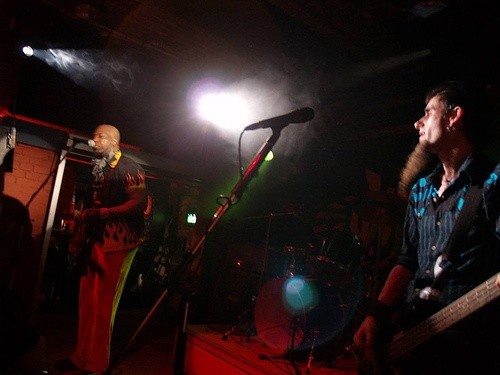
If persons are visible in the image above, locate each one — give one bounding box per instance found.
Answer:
[354,79,499,375]
[54,124,147,375]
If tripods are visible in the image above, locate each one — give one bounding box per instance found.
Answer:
[221,210,345,375]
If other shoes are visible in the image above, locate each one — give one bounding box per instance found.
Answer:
[47,358,82,375]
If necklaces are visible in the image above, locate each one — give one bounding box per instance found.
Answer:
[443,174,453,184]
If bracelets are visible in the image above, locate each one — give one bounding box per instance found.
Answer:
[368,300,388,324]
[98,208,109,220]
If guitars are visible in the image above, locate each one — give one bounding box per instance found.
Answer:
[354,272,500,374]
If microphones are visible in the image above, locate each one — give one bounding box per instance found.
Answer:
[72,137,95,147]
[244,107,315,130]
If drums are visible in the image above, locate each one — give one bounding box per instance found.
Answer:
[255,276,337,357]
